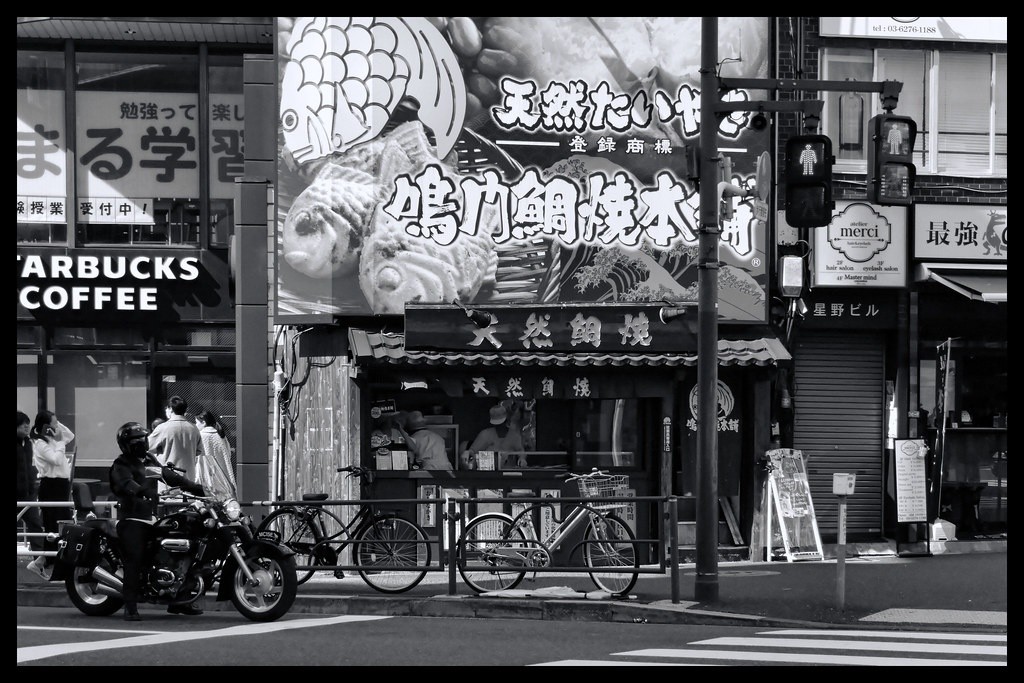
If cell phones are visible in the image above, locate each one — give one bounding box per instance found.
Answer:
[42,424,57,437]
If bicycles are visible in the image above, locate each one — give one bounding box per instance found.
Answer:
[455,467,641,595]
[255,466,432,595]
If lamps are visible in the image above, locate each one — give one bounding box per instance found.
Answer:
[452,298,492,329]
[781,389,791,409]
[660,298,687,324]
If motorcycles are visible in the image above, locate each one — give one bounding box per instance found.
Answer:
[54,460,298,623]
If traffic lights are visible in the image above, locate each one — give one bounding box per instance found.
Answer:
[867,111,918,206]
[784,134,836,229]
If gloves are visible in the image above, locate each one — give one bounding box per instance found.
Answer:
[136,485,161,503]
[192,485,206,497]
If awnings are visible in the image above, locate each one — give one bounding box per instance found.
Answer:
[348,327,791,399]
[933,274,1007,304]
[145,395,238,501]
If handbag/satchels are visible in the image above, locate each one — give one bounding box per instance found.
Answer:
[60,524,97,568]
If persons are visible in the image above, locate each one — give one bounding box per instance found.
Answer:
[397,412,453,470]
[108,423,207,620]
[17,412,46,561]
[28,410,75,580]
[461,405,526,468]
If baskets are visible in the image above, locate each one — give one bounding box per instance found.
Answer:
[576,475,630,510]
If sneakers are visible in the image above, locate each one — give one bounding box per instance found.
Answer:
[27,561,50,581]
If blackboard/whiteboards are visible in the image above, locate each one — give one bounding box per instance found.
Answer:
[768,448,824,561]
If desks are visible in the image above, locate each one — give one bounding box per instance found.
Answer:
[72,479,102,485]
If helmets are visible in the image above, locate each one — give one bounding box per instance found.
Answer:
[117,422,149,456]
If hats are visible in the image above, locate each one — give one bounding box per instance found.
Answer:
[405,411,427,431]
[489,406,507,425]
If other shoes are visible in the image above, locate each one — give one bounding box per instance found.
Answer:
[167,601,204,615]
[124,601,142,621]
